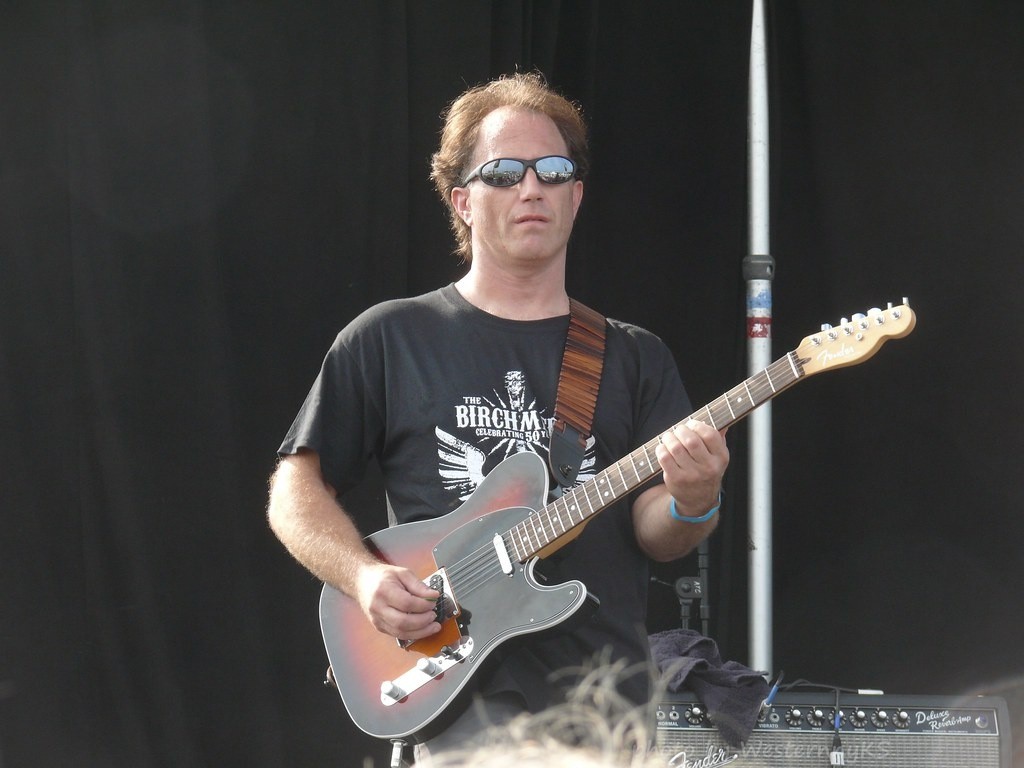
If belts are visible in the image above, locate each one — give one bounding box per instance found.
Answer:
[460,154,577,187]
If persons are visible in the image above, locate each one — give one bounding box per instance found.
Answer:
[264,76,731,767]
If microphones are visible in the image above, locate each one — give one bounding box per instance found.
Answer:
[830,734,845,768]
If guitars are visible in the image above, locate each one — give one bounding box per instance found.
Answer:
[313,290,920,747]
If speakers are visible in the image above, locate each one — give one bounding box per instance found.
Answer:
[651,689,1013,768]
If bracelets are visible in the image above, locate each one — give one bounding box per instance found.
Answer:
[671,491,721,523]
